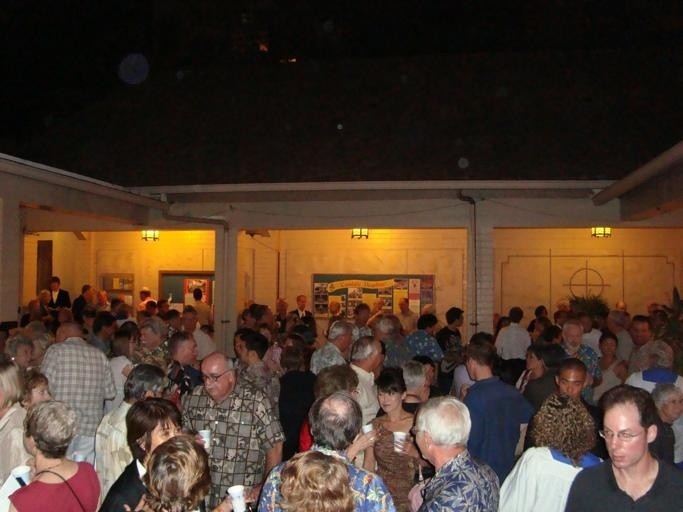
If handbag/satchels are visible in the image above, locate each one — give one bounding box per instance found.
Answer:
[406,464,431,512]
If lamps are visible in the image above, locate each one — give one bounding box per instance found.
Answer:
[141,231,160,242]
[351,229,369,239]
[591,227,611,238]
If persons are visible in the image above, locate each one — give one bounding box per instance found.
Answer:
[2,278,683,511]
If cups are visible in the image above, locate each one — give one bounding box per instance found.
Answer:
[71,449,88,462]
[199,429,210,449]
[361,422,374,443]
[225,484,247,512]
[393,430,407,454]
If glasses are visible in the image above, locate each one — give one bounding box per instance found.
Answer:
[201,371,228,382]
[598,430,642,442]
